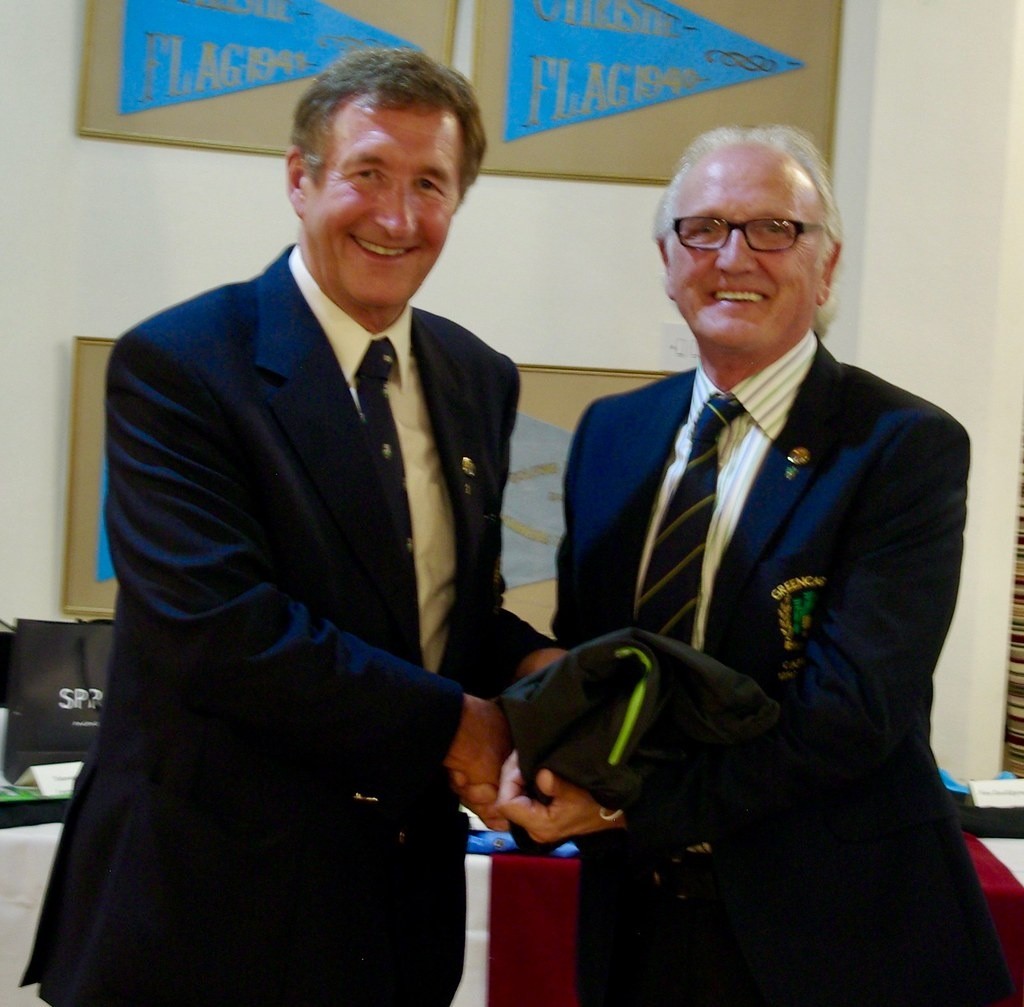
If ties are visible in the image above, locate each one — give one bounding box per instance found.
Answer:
[354,336,419,619]
[633,392,748,649]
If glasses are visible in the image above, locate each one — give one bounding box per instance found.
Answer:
[660,216,828,253]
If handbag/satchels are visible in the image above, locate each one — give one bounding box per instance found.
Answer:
[1,616,117,786]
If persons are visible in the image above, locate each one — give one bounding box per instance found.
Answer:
[445,128,1022,1006]
[20,49,575,1006]
